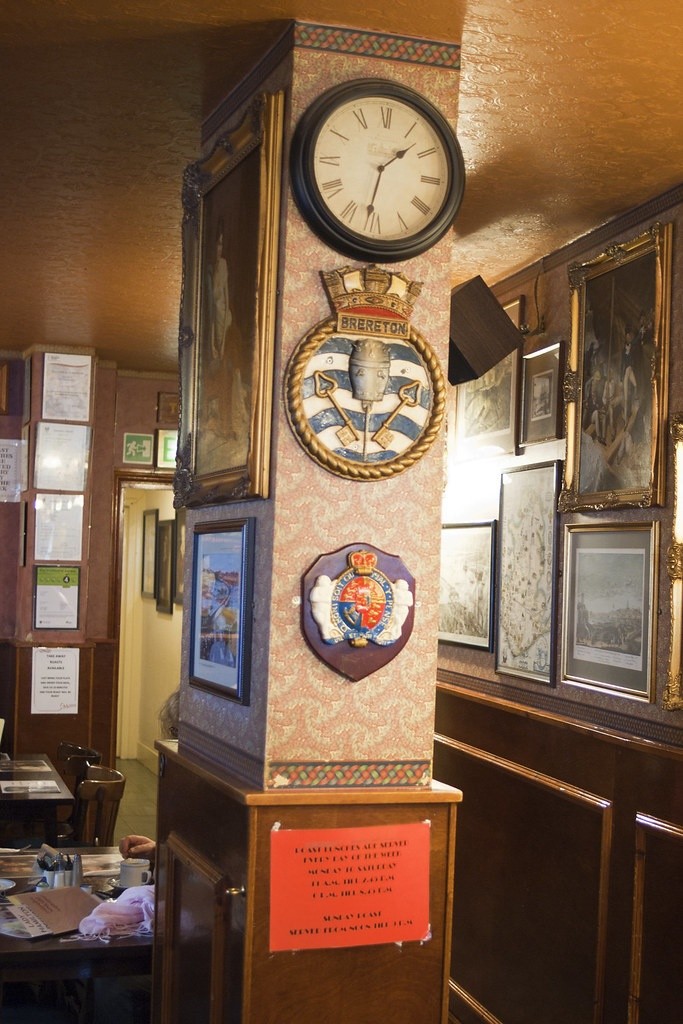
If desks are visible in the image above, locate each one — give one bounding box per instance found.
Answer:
[0,846,152,1024]
[0,753,76,848]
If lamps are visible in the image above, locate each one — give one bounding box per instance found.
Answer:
[447,275,524,387]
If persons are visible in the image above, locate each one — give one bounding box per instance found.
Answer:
[119,682,180,863]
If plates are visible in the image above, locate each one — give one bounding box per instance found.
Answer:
[108,875,128,889]
[0,878,16,890]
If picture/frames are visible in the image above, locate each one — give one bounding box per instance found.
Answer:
[519,340,565,449]
[556,221,673,516]
[187,516,255,706]
[141,508,159,600]
[172,87,285,510]
[161,831,229,1024]
[560,519,663,704]
[438,519,496,654]
[155,519,176,614]
[454,293,525,465]
[493,458,561,689]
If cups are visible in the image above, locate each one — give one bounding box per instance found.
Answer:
[120,858,152,886]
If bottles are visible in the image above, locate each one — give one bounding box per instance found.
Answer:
[34,853,82,896]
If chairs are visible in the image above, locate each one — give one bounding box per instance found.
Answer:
[6,740,103,846]
[8,763,127,847]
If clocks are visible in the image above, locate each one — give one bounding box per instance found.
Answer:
[288,79,467,264]
